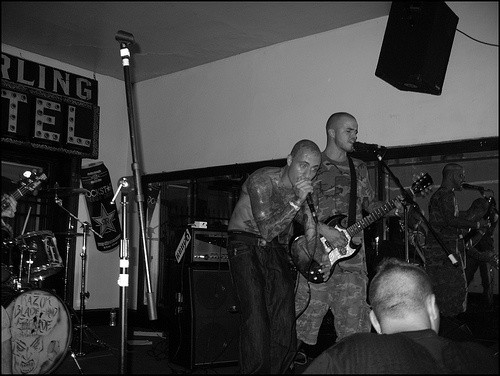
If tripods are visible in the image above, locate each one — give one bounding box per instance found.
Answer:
[52,197,120,358]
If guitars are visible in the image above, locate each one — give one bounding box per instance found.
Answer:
[1,168,48,215]
[289,172,435,284]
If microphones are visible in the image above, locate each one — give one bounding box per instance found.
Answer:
[352,141,388,152]
[306,192,318,224]
[462,184,490,191]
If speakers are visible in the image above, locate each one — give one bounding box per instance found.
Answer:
[169,266,241,369]
[375,0,460,96]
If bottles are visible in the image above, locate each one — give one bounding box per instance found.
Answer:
[174,289,183,315]
[109,306,116,326]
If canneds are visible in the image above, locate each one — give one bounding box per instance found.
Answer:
[81,161,123,252]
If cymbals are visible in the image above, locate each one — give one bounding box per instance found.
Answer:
[44,187,77,198]
[54,231,91,238]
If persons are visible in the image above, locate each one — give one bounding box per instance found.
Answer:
[229,139,320,375]
[395,208,401,218]
[427,162,491,338]
[292,113,405,363]
[303,258,500,376]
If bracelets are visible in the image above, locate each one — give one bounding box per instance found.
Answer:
[287,200,302,211]
[475,221,481,231]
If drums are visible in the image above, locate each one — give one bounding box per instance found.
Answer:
[11,230,66,281]
[1,285,72,374]
